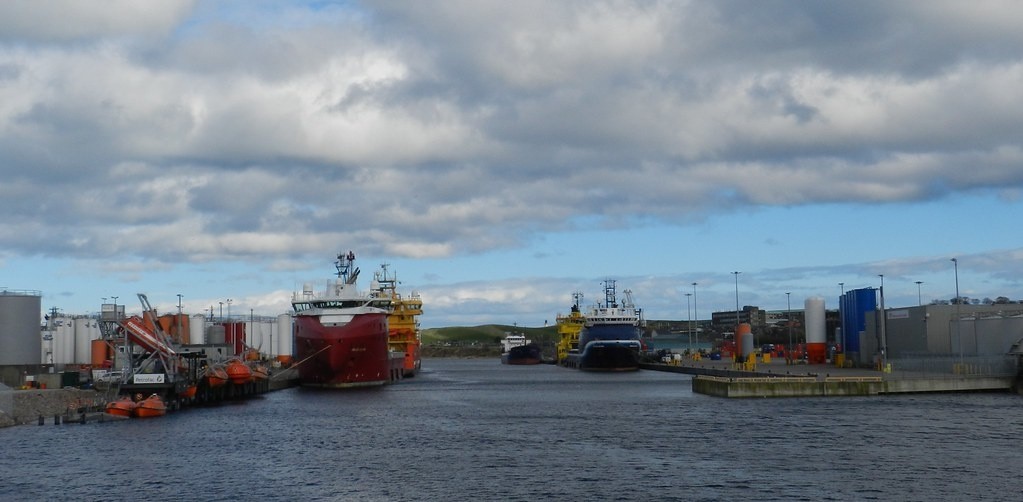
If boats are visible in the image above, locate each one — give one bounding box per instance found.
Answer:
[106,397,136,417]
[135,393,168,418]
[175,385,201,399]
[564,279,650,372]
[555,289,588,368]
[206,369,228,386]
[365,260,424,379]
[224,358,252,386]
[250,365,270,383]
[500,321,540,365]
[290,249,409,391]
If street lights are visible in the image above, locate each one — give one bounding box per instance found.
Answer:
[950,257,964,379]
[218,302,224,327]
[101,298,108,304]
[877,274,887,370]
[226,299,233,324]
[177,294,185,347]
[730,271,742,327]
[692,282,701,352]
[839,282,846,357]
[915,280,924,307]
[685,293,694,357]
[785,291,793,365]
[110,296,119,340]
